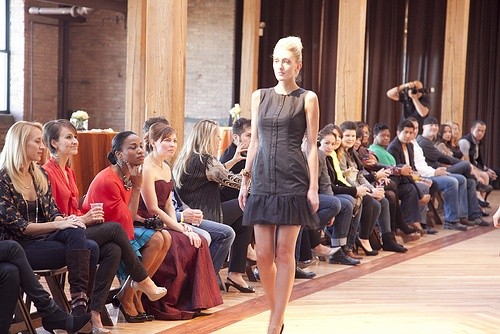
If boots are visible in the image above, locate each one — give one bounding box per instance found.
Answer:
[393,190,417,236]
[65,249,92,334]
[329,246,360,265]
[88,266,97,313]
[390,206,404,247]
[382,232,405,252]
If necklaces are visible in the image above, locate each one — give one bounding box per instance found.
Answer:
[117,162,132,191]
[154,161,163,169]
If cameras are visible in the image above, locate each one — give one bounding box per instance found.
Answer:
[412,86,430,94]
[143,215,163,229]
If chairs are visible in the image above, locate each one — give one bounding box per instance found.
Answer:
[17,265,114,334]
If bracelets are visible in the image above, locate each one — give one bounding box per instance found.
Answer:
[240,169,252,178]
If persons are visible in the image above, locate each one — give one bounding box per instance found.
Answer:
[237,36,320,334]
[0,116,498,334]
[492,207,500,228]
[387,80,432,135]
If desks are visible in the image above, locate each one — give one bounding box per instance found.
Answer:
[39,131,119,198]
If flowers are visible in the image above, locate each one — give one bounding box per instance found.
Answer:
[72,109,90,122]
[230,103,241,124]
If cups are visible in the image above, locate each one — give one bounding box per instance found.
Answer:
[349,170,359,181]
[90,203,104,224]
[376,186,384,196]
[192,210,201,225]
[413,172,420,181]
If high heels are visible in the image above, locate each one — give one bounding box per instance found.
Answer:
[296,256,319,269]
[91,327,110,334]
[311,244,341,261]
[225,277,255,293]
[137,310,154,321]
[112,295,146,323]
[419,194,431,207]
[398,228,421,243]
[42,307,92,334]
[245,257,256,264]
[130,279,167,301]
[355,237,378,255]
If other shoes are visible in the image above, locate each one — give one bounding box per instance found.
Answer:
[279,324,284,334]
[460,217,475,226]
[295,267,316,279]
[421,222,438,233]
[345,249,363,259]
[477,198,489,207]
[468,216,489,226]
[410,222,428,234]
[443,221,467,230]
[482,211,489,216]
